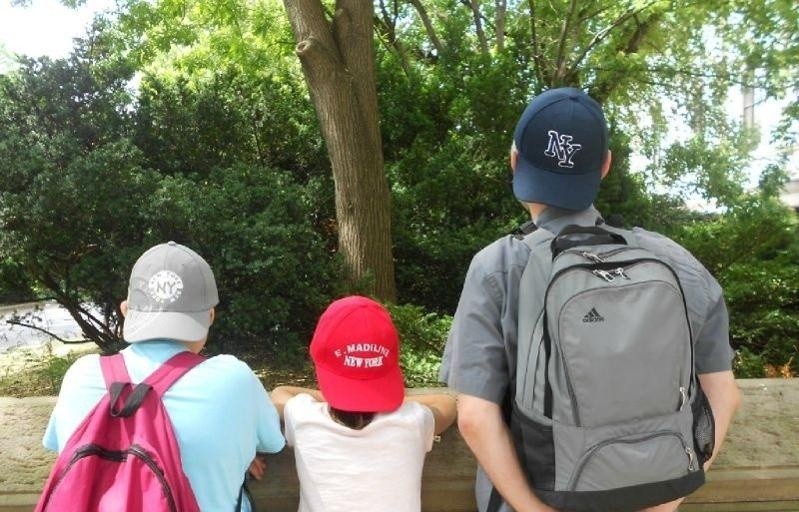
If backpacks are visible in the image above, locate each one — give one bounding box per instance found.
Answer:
[508,222,714,511]
[34,351,207,511]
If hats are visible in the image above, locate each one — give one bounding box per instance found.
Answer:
[122,241,219,343]
[309,296,404,413]
[513,87,609,212]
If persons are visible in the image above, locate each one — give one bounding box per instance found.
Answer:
[436,86,740,512]
[269,294,458,512]
[42,240,286,512]
